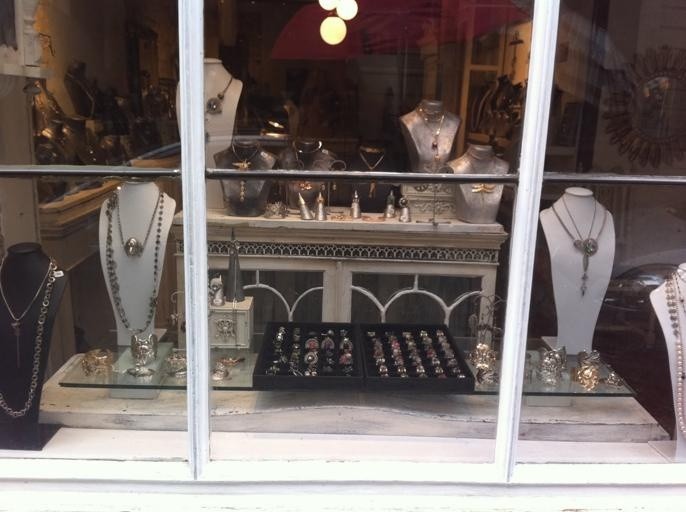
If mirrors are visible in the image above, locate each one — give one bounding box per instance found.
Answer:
[600,47,686,167]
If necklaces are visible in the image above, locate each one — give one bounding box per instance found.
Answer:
[358,150,387,199]
[205,76,236,145]
[414,105,449,168]
[103,190,166,334]
[1,256,60,418]
[229,140,261,203]
[549,201,609,298]
[291,141,325,192]
[1,258,53,368]
[662,266,686,438]
[114,187,161,261]
[463,152,499,207]
[560,194,600,255]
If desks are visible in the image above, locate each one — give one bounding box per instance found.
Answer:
[170,202,508,358]
[38,349,670,442]
